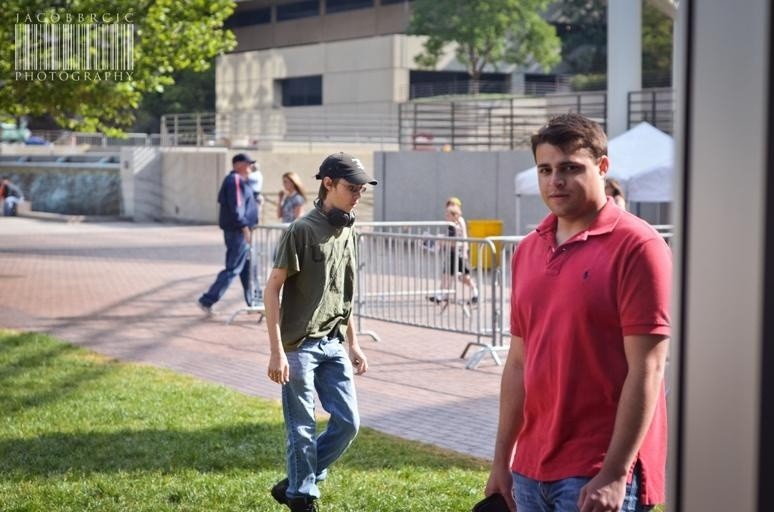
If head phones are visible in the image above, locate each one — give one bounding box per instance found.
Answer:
[314,197,356,228]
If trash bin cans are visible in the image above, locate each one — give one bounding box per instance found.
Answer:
[466,218,503,268]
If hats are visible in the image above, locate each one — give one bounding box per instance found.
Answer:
[232,153,256,165]
[315,150,378,187]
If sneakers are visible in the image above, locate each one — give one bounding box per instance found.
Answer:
[467,288,480,306]
[288,495,319,512]
[195,297,219,317]
[271,478,290,507]
[430,296,445,304]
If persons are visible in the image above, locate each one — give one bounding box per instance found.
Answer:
[485,109,673,512]
[247,164,262,203]
[604,178,628,213]
[277,171,308,225]
[427,207,480,306]
[195,154,265,314]
[264,152,378,512]
[1,174,25,216]
[445,196,469,262]
